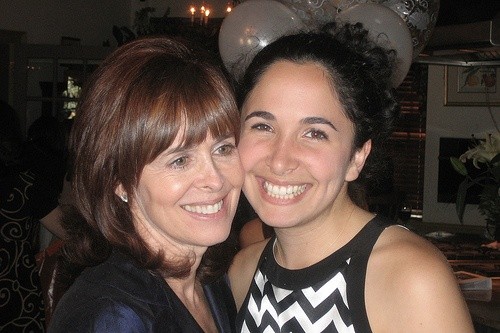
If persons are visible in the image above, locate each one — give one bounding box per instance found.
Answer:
[1,98,73,333]
[229,32,475,333]
[241,189,369,249]
[48,40,239,333]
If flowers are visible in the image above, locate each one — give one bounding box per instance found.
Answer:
[450,128,500,239]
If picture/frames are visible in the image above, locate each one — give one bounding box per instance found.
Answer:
[444,65,500,107]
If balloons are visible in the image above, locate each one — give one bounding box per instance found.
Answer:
[219,0,441,90]
